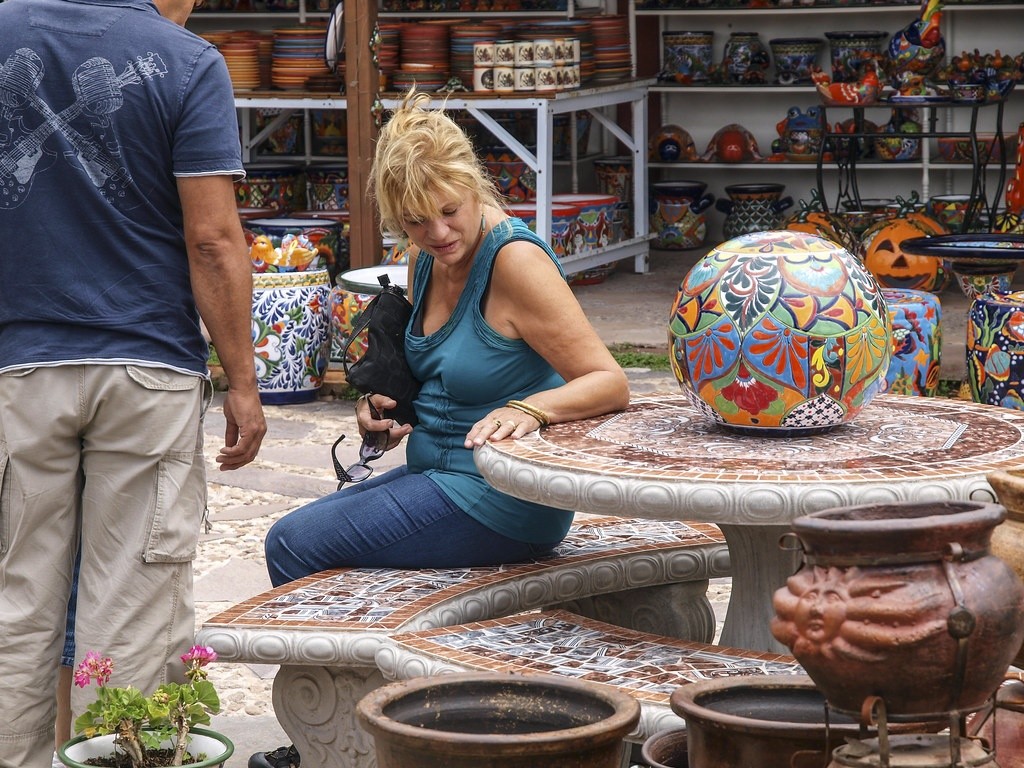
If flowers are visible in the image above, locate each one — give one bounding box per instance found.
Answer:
[72,644,222,768]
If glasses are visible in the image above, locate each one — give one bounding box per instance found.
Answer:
[331,396,390,483]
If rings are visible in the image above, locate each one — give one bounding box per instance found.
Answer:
[494,419,501,427]
[507,420,516,430]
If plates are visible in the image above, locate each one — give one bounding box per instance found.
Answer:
[195,11,631,94]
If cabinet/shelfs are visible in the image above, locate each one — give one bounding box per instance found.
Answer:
[626,0,1024,171]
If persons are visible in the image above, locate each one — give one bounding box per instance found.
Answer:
[262,79,632,584]
[0,0,267,768]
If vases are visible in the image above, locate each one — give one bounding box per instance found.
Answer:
[57,728,234,768]
[769,495,1023,718]
[661,25,1017,297]
[663,674,946,767]
[986,467,1023,587]
[642,725,691,768]
[358,671,638,767]
[234,110,630,405]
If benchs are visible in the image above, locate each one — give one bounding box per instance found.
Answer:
[196,516,1024,768]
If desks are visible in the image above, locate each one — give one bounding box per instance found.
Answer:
[336,265,408,296]
[231,77,653,282]
[467,379,1022,656]
[811,97,1005,236]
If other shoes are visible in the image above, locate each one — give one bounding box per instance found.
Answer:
[248,742,301,768]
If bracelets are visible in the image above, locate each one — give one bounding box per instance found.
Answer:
[504,400,552,427]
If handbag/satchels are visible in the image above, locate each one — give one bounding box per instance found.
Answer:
[341,271,423,429]
